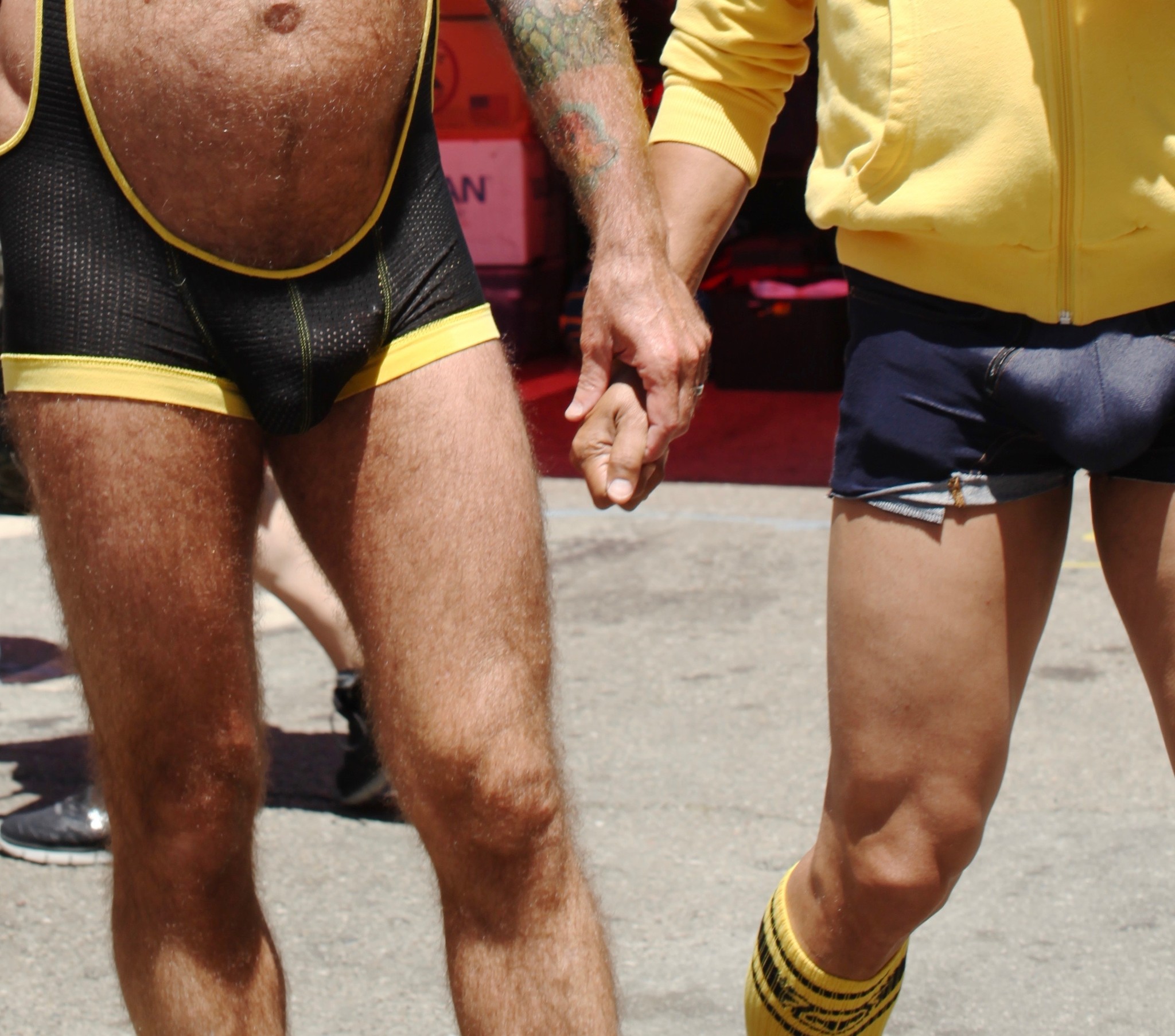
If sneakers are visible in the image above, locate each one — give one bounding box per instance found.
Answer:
[331,669,392,806]
[0,781,118,866]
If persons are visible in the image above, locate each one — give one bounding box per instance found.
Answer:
[570,0,1175,1036]
[0,1,712,1036]
[0,456,392,865]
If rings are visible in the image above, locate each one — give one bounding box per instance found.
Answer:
[692,383,704,396]
